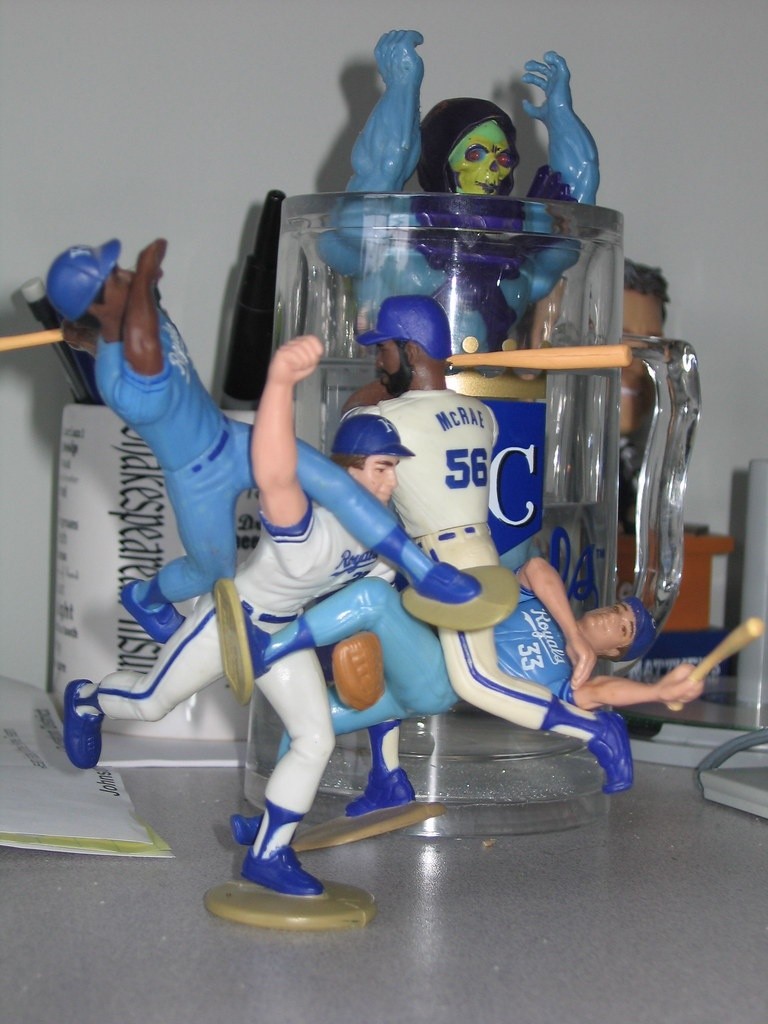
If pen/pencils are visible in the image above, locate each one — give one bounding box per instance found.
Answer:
[19,276,97,404]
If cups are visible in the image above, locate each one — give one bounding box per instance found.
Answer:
[53,399,279,741]
[243,190,702,835]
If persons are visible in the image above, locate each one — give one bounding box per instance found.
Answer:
[46,237,703,904]
[319,28,603,380]
[619,261,669,536]
